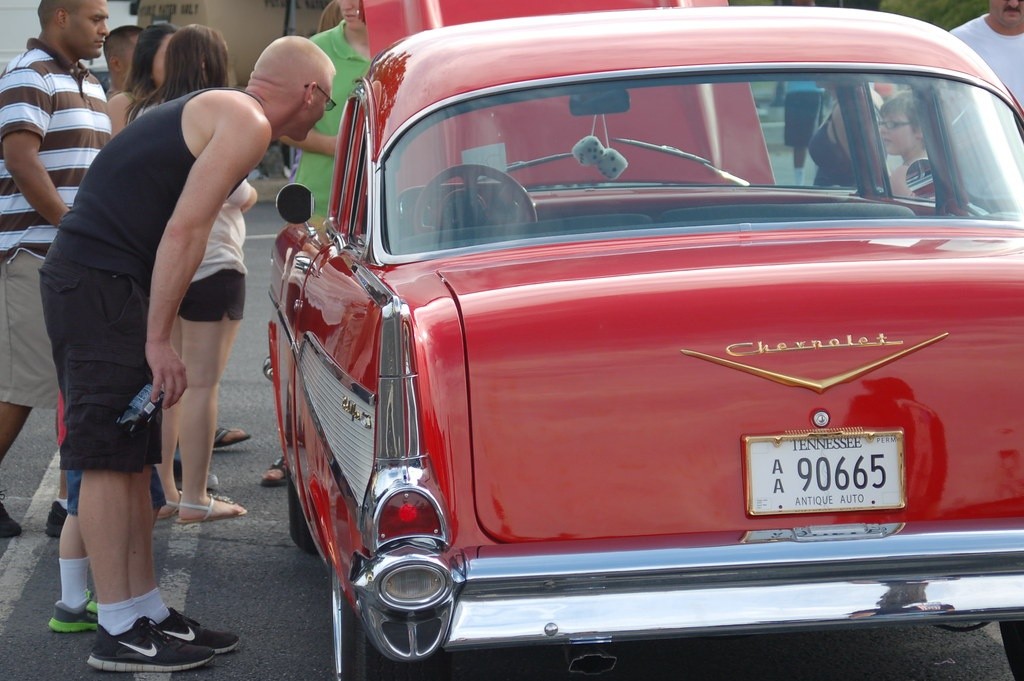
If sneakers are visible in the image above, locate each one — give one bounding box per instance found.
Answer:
[150,607,240,655]
[46,501,68,538]
[48,590,99,632]
[0,489,21,537]
[87,615,216,672]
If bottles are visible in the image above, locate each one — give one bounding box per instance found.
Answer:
[117,378,163,433]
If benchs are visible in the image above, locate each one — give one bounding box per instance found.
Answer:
[400,202,915,247]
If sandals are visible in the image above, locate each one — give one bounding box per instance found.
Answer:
[175,494,247,524]
[214,426,250,448]
[156,490,183,520]
[261,456,288,487]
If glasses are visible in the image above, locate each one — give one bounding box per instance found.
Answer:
[304,83,336,111]
[878,119,912,129]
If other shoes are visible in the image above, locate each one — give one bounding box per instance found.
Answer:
[206,474,218,490]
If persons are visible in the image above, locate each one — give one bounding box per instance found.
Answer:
[809,81,856,187]
[43,36,336,672]
[949,0,1024,107]
[261,0,371,485]
[101,23,257,525]
[0,0,113,539]
[783,80,826,185]
[49,465,166,632]
[879,92,935,200]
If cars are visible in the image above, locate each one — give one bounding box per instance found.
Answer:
[265,0,1024,680]
